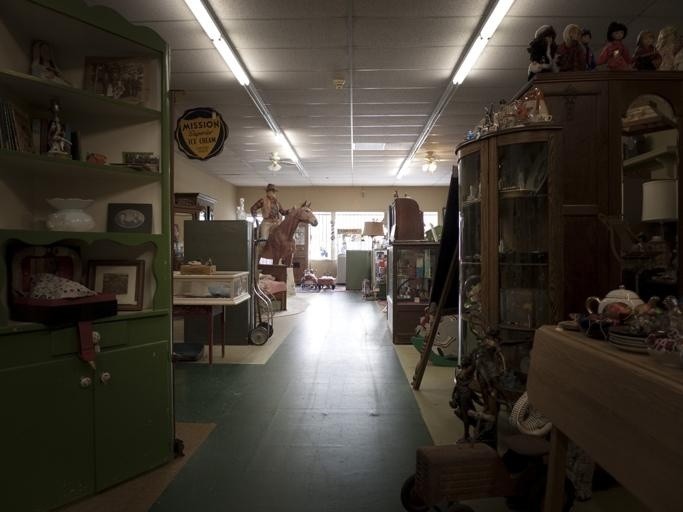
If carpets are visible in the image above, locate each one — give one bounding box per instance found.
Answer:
[374,298,388,320]
[391,333,644,507]
[52,419,218,511]
[253,296,309,318]
[173,317,298,365]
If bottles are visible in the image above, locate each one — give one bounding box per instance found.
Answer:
[46,209,94,233]
[236,197,246,221]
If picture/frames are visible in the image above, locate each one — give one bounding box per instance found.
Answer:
[104,203,153,234]
[83,255,144,314]
[83,49,150,107]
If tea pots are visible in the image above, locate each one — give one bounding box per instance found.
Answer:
[583,282,644,314]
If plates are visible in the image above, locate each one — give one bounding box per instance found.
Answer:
[607,329,648,354]
[499,189,528,197]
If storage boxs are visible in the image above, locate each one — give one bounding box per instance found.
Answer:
[3,235,117,330]
[498,284,552,328]
[170,267,250,299]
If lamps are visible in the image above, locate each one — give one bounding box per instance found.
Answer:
[267,161,281,171]
[360,221,386,300]
[421,150,437,173]
[638,179,678,280]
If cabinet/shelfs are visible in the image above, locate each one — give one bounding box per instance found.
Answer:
[1,1,178,511]
[388,240,442,345]
[285,215,311,283]
[386,197,424,243]
[175,190,215,225]
[457,124,566,368]
[508,68,680,323]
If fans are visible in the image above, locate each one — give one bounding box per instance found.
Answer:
[247,151,297,167]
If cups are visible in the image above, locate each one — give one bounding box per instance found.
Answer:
[85,153,105,165]
[534,113,552,122]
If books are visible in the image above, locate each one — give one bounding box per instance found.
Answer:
[0,103,36,152]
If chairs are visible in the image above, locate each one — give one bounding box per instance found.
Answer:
[256,273,287,312]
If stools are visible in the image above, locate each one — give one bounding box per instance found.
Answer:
[318,275,336,290]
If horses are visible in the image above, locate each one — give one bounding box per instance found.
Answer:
[253,200,319,265]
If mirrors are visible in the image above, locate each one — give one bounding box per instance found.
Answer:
[616,90,681,308]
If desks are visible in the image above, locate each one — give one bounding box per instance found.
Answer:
[528,323,682,512]
[168,292,251,367]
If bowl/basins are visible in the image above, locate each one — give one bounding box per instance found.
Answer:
[646,345,682,368]
[45,197,92,210]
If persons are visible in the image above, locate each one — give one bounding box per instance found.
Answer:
[251,184,289,265]
[528,22,662,80]
